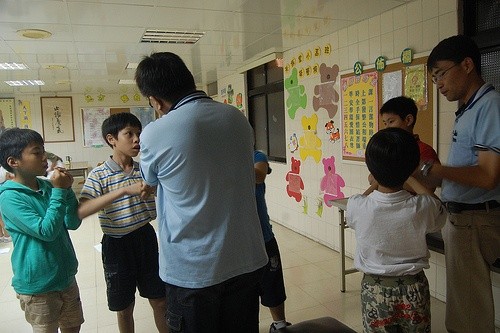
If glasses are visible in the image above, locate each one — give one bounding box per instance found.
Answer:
[148,98,153,108]
[431,63,460,84]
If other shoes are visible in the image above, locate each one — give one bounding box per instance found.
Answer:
[269,322,292,333]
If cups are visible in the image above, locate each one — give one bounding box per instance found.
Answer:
[66,156,72,162]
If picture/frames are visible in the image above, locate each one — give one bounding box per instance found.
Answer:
[40,95,75,143]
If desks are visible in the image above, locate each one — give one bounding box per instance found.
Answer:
[329,197,500,293]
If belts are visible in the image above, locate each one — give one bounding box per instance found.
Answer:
[444,200,500,212]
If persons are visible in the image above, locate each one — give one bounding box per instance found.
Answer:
[377,97,444,234]
[250,148,293,333]
[0,152,59,243]
[76,111,170,333]
[427,33,500,333]
[346,128,447,333]
[0,127,85,333]
[136,49,270,333]
